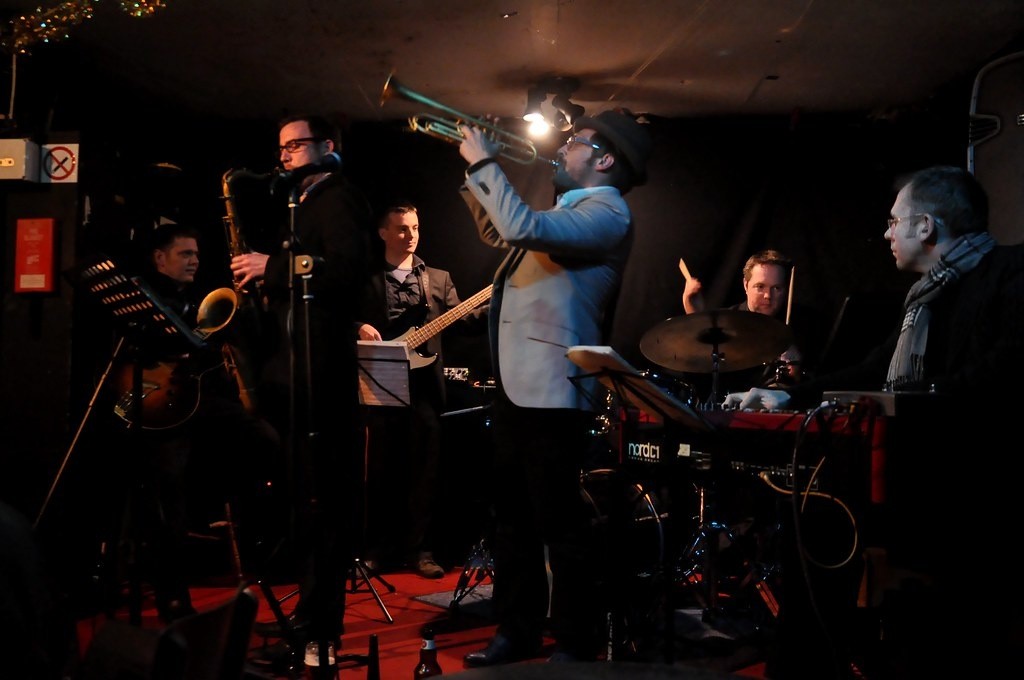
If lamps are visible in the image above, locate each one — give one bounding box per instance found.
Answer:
[523,81,584,139]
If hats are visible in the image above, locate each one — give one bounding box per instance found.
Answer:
[574,111,651,187]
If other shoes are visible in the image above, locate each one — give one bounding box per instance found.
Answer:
[355,560,374,577]
[419,557,445,577]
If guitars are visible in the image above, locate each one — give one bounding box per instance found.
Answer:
[359,283,495,371]
[110,317,234,435]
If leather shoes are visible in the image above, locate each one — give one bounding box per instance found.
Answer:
[254,608,342,657]
[464,635,542,667]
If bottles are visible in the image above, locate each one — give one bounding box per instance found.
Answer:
[414,630,441,680]
[304,631,336,680]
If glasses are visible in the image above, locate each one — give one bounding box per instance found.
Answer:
[887,213,943,232]
[279,137,315,152]
[567,137,603,151]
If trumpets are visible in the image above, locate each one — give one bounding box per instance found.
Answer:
[372,64,564,171]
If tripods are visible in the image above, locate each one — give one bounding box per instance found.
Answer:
[274,358,413,625]
[650,449,782,632]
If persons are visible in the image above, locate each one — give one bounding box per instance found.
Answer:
[340,202,490,578]
[764,164,1024,680]
[222,115,355,668]
[456,111,650,667]
[680,247,802,406]
[134,224,243,343]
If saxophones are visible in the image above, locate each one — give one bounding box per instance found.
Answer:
[195,166,295,405]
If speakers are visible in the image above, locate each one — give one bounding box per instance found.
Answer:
[966,51,1024,247]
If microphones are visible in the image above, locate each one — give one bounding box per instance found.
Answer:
[278,151,347,184]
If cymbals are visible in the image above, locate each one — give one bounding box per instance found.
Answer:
[639,307,793,375]
